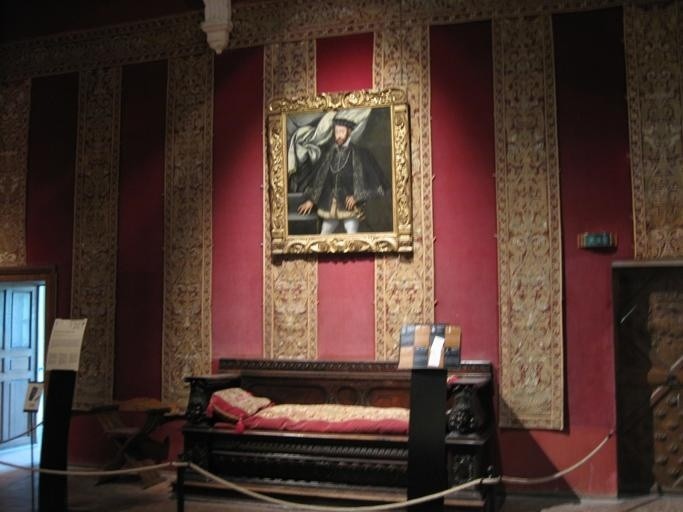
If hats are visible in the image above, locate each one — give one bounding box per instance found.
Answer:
[330,117,358,132]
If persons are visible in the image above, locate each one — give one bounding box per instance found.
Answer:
[295,116,389,235]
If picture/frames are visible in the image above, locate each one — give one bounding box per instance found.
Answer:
[268,87,414,258]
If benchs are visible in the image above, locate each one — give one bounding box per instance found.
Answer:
[180,357,508,512]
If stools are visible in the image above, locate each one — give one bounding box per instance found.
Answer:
[84,404,171,491]
